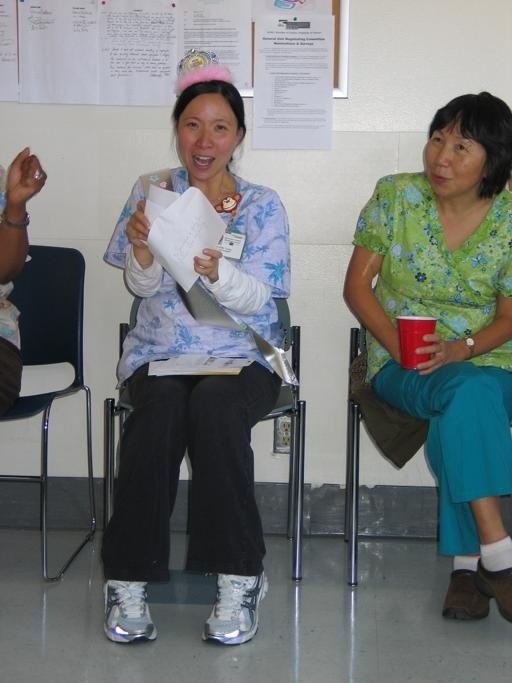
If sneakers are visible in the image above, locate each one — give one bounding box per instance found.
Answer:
[442,571,490,618]
[203,571,268,645]
[104,581,157,644]
[476,558,512,621]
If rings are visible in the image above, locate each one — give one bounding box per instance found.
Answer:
[30,167,44,182]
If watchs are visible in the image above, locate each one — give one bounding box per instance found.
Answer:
[460,334,477,361]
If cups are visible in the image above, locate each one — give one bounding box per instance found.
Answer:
[395,313,438,370]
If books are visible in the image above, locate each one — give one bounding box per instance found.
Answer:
[143,352,247,378]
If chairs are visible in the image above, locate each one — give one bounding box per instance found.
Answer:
[0,243,99,583]
[344,284,433,590]
[100,299,305,583]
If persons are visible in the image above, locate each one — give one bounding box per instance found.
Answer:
[0,145,48,412]
[101,48,293,648]
[341,91,512,625]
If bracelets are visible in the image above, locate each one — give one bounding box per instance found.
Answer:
[2,206,31,227]
